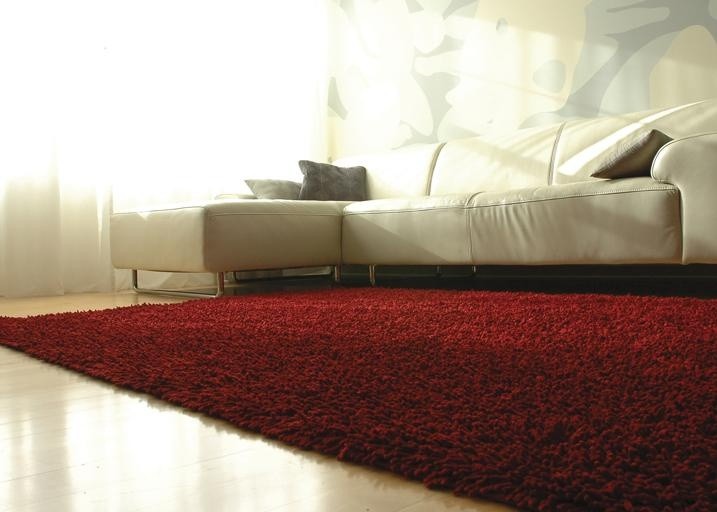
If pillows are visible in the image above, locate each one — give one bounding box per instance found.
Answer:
[243,159,368,200]
[589,129,670,179]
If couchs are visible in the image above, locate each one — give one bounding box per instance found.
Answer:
[108,98,717,301]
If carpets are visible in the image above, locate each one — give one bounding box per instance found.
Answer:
[0,283,717,512]
